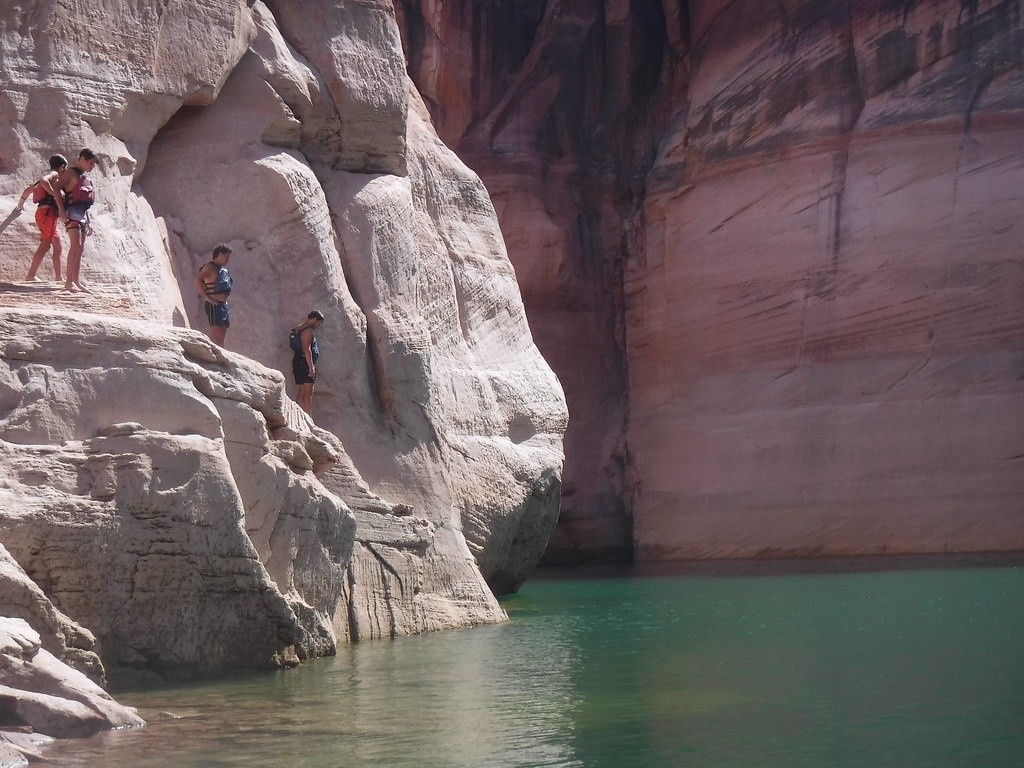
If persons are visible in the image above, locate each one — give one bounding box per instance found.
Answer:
[194,243,232,346]
[289,310,323,420]
[55,148,100,294]
[18,154,64,282]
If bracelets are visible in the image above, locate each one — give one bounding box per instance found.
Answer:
[21,196,26,200]
